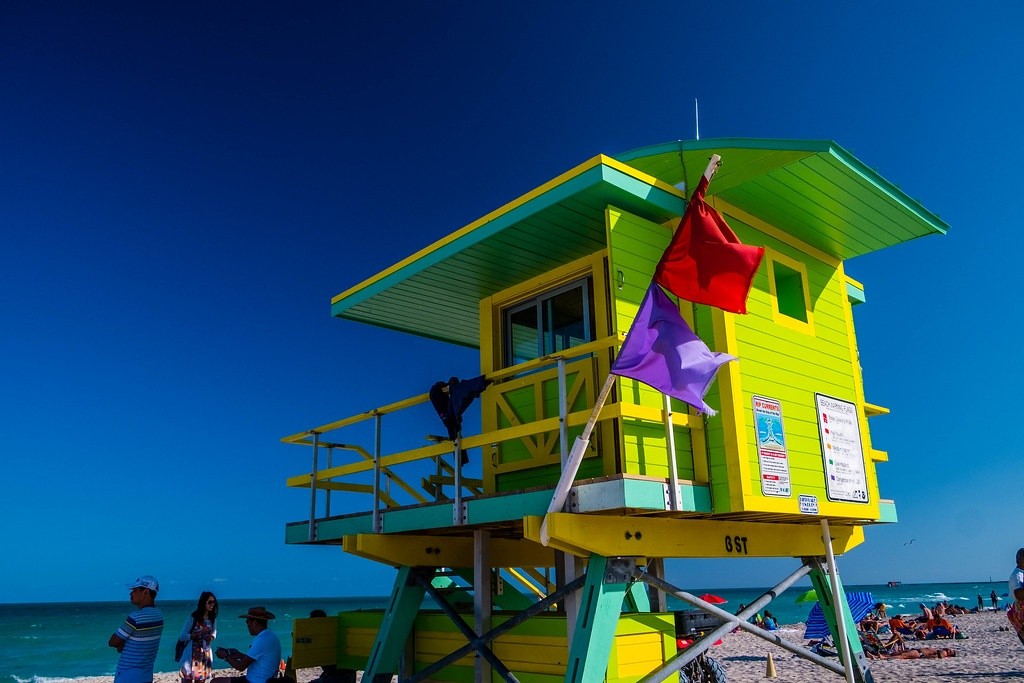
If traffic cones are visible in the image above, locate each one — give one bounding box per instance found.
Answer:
[764,652,778,678]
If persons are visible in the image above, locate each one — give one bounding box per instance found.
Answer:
[977,594,983,610]
[176,592,219,683]
[108,575,164,683]
[1009,548,1024,603]
[990,591,998,608]
[759,610,778,631]
[914,603,934,623]
[737,604,758,626]
[310,610,356,683]
[867,648,956,660]
[210,606,281,683]
[861,600,978,652]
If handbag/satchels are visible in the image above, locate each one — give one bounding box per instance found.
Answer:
[175,638,185,662]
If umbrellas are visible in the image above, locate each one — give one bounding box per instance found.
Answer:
[803,592,876,639]
[796,589,818,602]
[700,593,727,604]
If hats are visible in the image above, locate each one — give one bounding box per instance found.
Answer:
[238,607,275,620]
[127,576,159,591]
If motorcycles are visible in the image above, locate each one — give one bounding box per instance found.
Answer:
[668,609,726,683]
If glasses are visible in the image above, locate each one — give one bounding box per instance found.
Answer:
[206,600,215,605]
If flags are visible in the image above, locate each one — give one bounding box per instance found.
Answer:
[655,177,765,314]
[610,283,739,413]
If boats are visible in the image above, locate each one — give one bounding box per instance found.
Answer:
[929,592,944,599]
[888,582,902,587]
